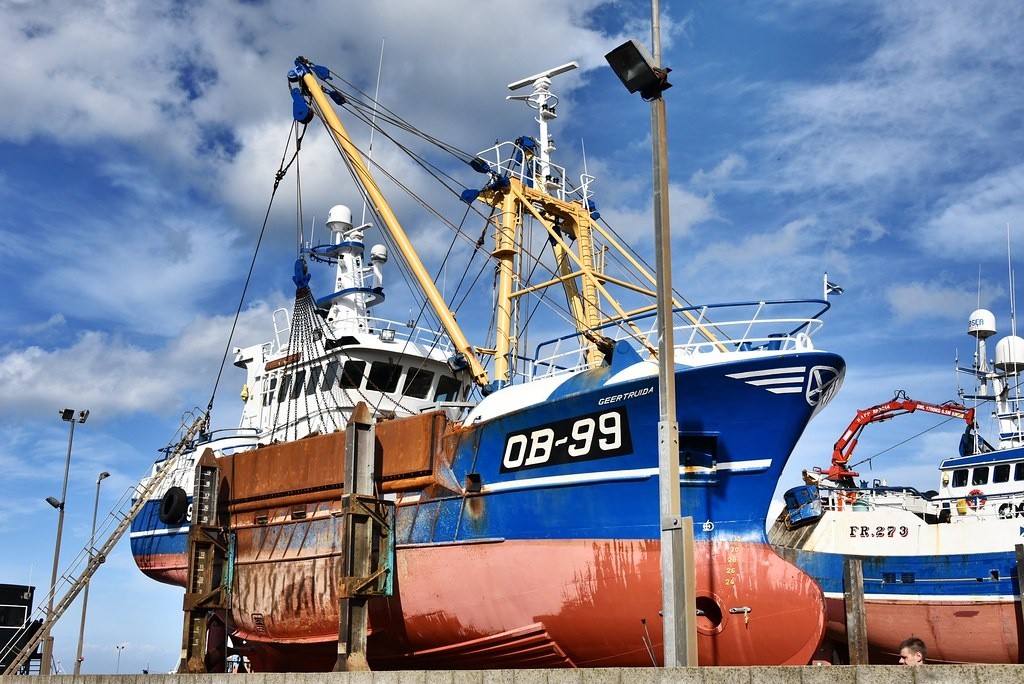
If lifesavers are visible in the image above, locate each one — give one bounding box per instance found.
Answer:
[999,499,1024,519]
[967,489,986,509]
[158,485,187,525]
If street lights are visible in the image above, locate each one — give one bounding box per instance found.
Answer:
[44,407,90,635]
[73,472,111,676]
[116,645,125,674]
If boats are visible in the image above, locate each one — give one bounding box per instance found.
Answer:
[126,52,846,675]
[766,218,1024,664]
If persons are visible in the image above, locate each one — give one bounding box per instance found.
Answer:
[898,638,927,666]
